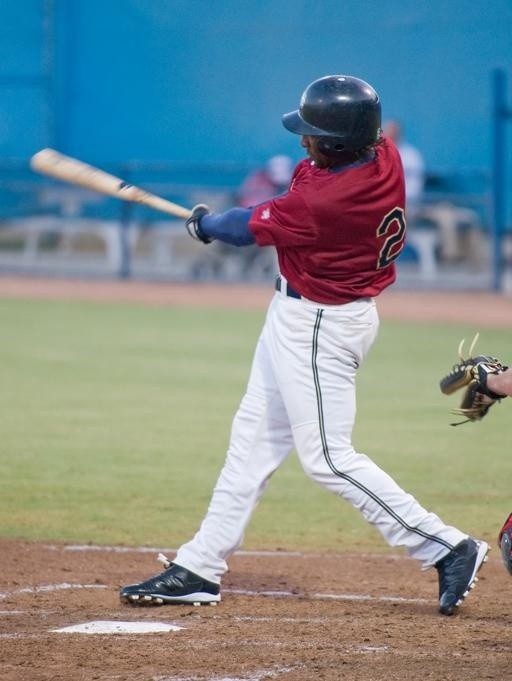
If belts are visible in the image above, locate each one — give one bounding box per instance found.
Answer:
[274,275,302,298]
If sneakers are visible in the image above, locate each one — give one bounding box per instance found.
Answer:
[436,538,491,614]
[121,562,221,605]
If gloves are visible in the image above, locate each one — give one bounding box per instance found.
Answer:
[183,205,219,243]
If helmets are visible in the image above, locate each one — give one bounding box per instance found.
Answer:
[283,75,382,155]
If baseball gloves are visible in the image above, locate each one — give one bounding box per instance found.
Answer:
[440,354,507,420]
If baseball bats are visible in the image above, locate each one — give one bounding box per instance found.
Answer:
[30,150,191,220]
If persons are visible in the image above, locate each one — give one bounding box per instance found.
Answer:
[237,121,427,277]
[439,354,512,575]
[119,75,492,615]
